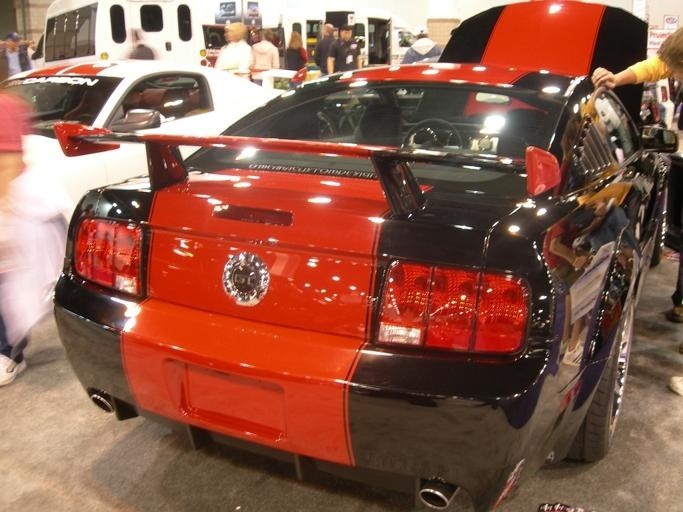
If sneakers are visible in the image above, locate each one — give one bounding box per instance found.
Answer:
[668,375,683,395]
[669,304,683,324]
[0,351,29,386]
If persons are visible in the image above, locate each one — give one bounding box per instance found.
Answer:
[314,24,336,76]
[401,24,442,65]
[665,373,683,398]
[0,32,34,77]
[286,31,307,70]
[214,22,253,81]
[250,30,282,72]
[591,20,682,322]
[0,88,28,390]
[326,24,362,75]
